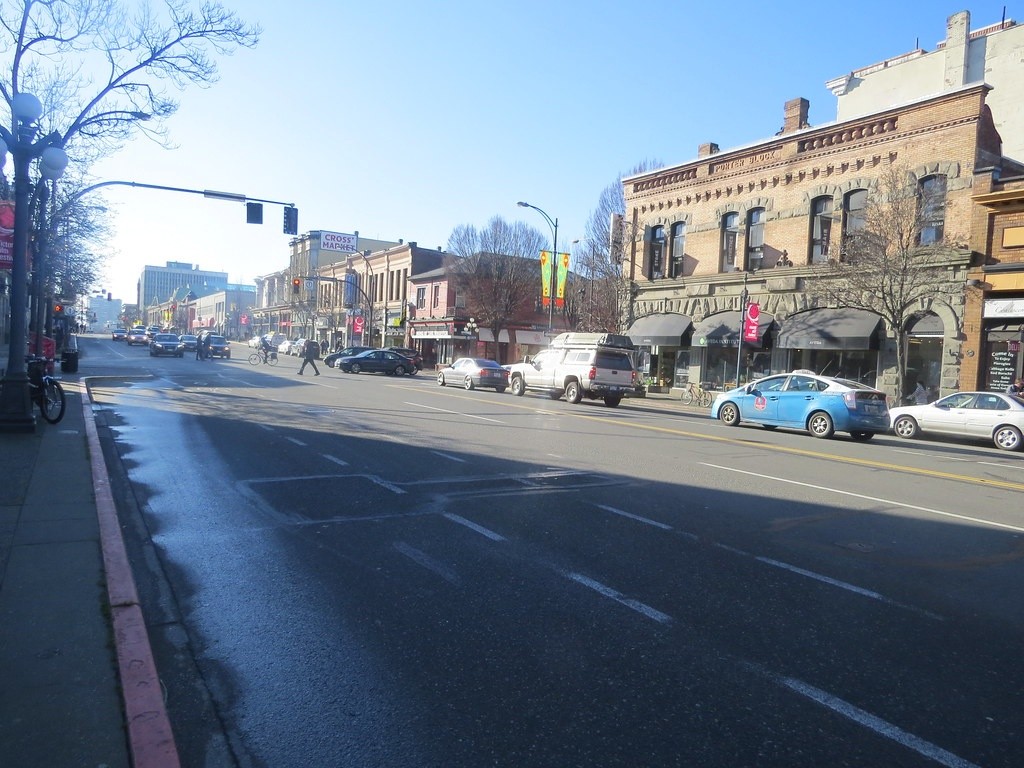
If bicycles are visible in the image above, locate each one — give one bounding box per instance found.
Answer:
[680,381,712,408]
[248,347,278,366]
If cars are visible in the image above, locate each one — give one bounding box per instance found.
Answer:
[126,324,162,346]
[322,345,425,378]
[247,331,321,361]
[178,334,198,352]
[436,357,511,394]
[196,328,231,360]
[710,368,891,443]
[149,333,185,358]
[111,329,128,342]
[887,391,1024,452]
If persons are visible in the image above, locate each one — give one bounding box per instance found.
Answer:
[196,334,206,360]
[906,380,928,405]
[259,337,269,365]
[74,322,86,335]
[334,337,343,353]
[296,339,320,376]
[203,333,211,359]
[1006,377,1024,398]
[321,337,329,355]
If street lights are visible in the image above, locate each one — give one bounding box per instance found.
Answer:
[337,245,374,347]
[572,238,595,332]
[0,92,70,434]
[463,317,480,357]
[516,201,558,344]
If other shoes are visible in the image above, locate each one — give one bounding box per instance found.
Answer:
[297,373,303,375]
[315,373,320,376]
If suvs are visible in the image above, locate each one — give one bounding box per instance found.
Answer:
[507,331,638,408]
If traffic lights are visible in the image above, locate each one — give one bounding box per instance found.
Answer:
[294,279,300,294]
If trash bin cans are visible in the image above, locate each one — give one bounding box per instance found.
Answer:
[61,348,80,373]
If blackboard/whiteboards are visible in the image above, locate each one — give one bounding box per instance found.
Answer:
[989,351,1017,392]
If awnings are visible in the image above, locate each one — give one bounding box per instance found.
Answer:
[479,326,509,343]
[777,307,882,350]
[625,312,691,347]
[515,330,551,345]
[691,310,773,349]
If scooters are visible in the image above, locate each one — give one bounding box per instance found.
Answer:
[24,353,67,425]
